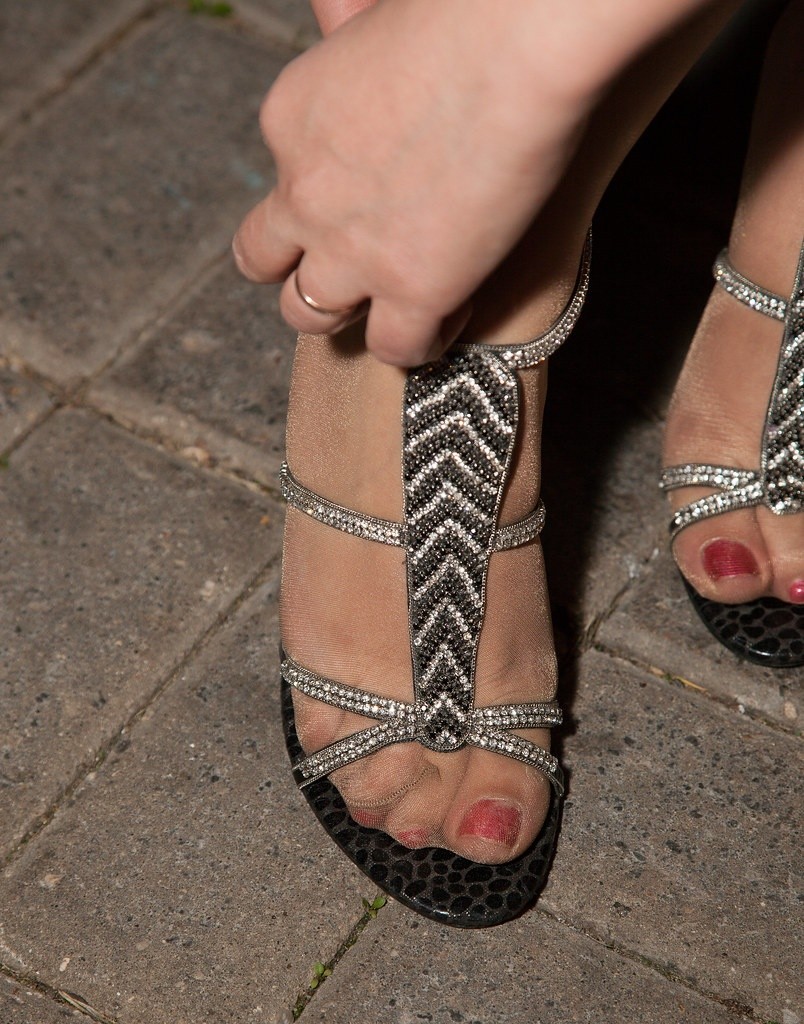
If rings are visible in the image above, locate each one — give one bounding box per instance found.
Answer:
[294,272,353,316]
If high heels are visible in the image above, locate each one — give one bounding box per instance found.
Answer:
[657,244,804,669]
[280,222,594,929]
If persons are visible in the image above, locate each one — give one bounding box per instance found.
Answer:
[231,0,804,926]
